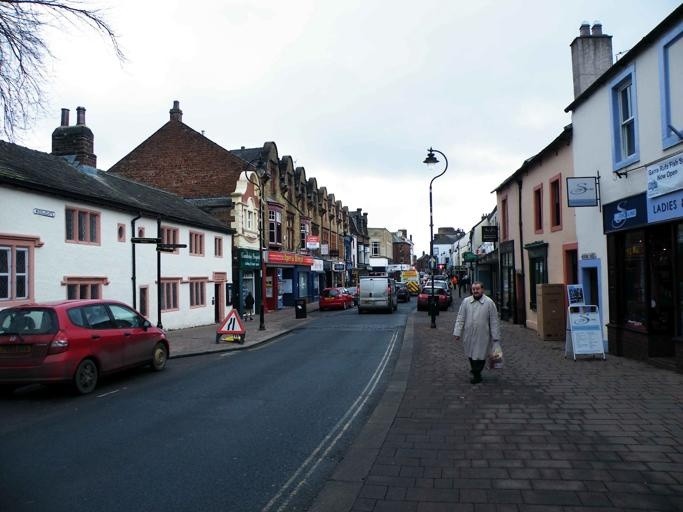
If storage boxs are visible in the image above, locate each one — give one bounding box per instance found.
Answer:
[535,283,566,343]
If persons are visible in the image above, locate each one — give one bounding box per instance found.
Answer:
[452,281,502,384]
[452,275,457,289]
[244,291,254,321]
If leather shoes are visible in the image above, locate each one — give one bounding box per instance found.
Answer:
[471,377,481,384]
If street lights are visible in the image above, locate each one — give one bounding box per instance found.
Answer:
[245,154,271,330]
[423,148,448,328]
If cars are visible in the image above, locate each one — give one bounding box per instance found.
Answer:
[317,268,452,314]
[0,298,170,396]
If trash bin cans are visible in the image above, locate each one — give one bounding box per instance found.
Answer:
[294,298,306,319]
